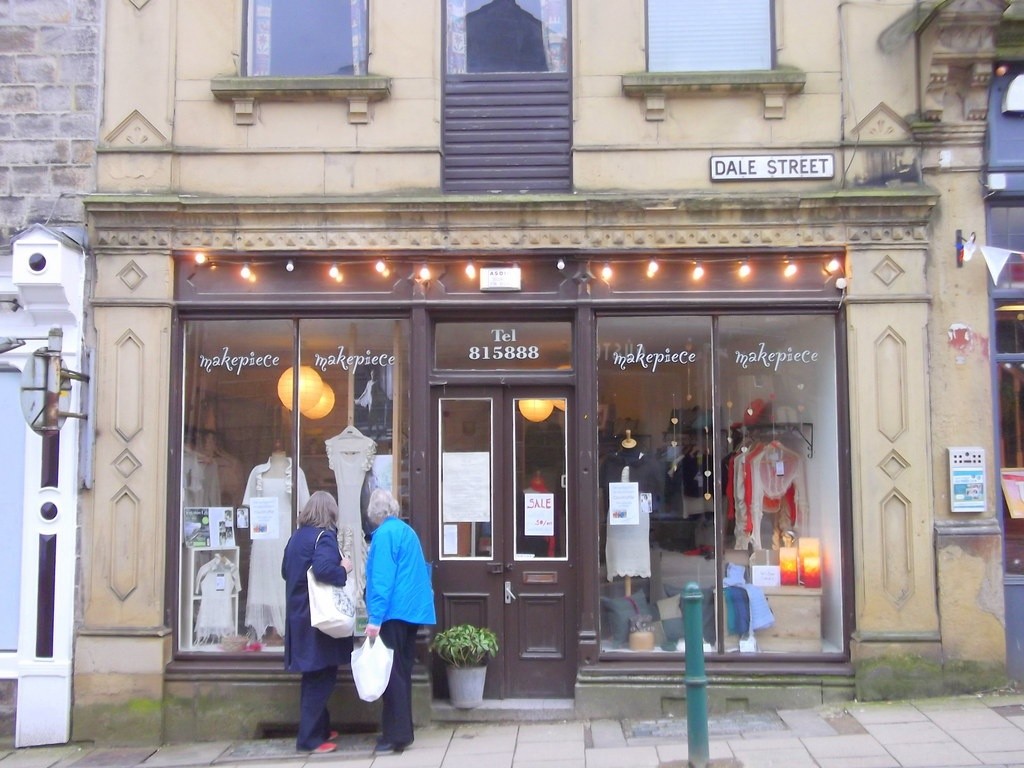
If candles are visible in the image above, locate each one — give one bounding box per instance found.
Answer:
[799,536,820,588]
[780,543,798,587]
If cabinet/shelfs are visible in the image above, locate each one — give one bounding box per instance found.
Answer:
[182,546,239,651]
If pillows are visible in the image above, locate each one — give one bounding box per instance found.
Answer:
[656,584,716,645]
[601,588,654,649]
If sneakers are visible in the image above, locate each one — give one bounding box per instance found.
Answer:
[325,731,337,741]
[297,743,337,754]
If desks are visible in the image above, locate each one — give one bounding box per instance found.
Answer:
[714,586,822,654]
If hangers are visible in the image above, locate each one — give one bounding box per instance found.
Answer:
[654,429,800,464]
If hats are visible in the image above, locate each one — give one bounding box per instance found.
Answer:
[744,400,769,425]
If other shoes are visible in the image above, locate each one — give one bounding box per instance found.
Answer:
[375,736,414,754]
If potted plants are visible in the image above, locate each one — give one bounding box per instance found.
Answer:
[432,625,498,708]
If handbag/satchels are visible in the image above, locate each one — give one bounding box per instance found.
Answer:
[306,567,358,638]
[352,634,394,701]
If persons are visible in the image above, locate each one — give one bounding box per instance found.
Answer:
[281,490,353,752]
[364,489,436,754]
[242,451,311,641]
[641,494,652,512]
[599,438,664,580]
